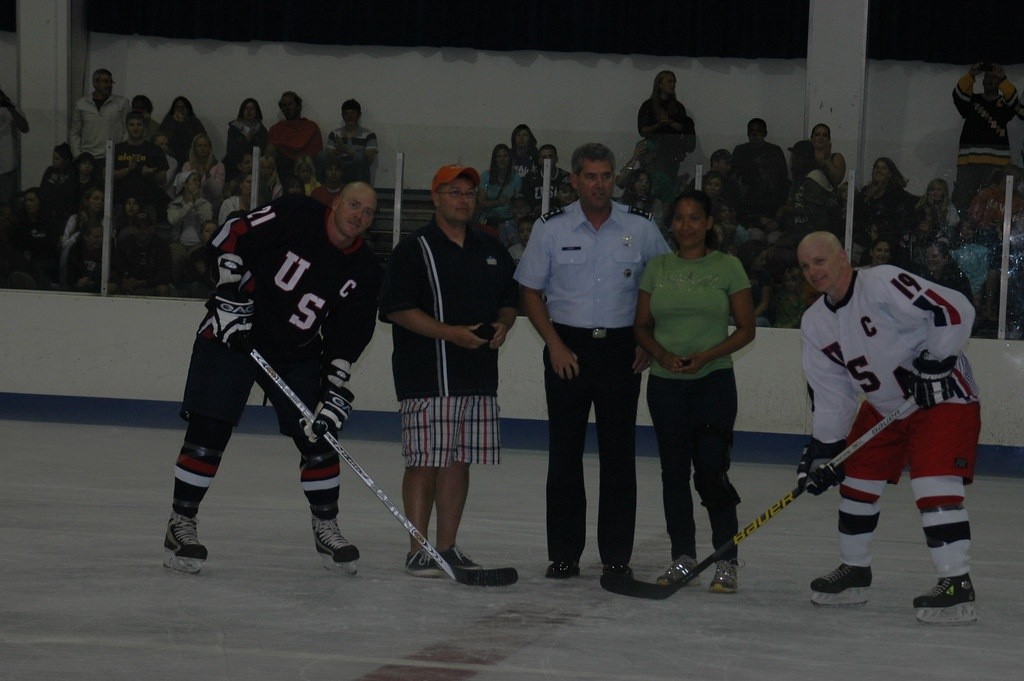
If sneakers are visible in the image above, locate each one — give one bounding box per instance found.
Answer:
[405,549,441,576]
[311,514,360,575]
[913,573,978,623]
[163,509,207,574]
[710,558,744,592]
[656,554,699,586]
[810,563,874,606]
[440,545,481,569]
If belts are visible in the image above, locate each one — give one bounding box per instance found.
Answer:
[553,321,635,338]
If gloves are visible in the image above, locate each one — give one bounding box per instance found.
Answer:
[211,296,254,352]
[299,384,355,442]
[797,434,848,494]
[912,349,956,407]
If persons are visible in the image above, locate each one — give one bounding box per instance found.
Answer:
[514,143,672,578]
[796,232,981,627]
[633,190,755,594]
[475,62,1024,341]
[379,165,519,577]
[0,67,377,298]
[161,181,380,575]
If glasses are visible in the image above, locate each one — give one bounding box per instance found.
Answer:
[440,189,478,199]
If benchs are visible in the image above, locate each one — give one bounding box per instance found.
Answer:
[366,190,436,266]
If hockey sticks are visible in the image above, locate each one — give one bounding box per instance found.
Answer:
[251,348,519,588]
[600,392,915,600]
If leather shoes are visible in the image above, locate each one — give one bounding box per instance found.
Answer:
[602,563,635,579]
[545,561,580,578]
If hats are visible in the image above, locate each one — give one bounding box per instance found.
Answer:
[132,212,154,226]
[174,170,197,193]
[431,164,480,193]
[787,140,813,159]
